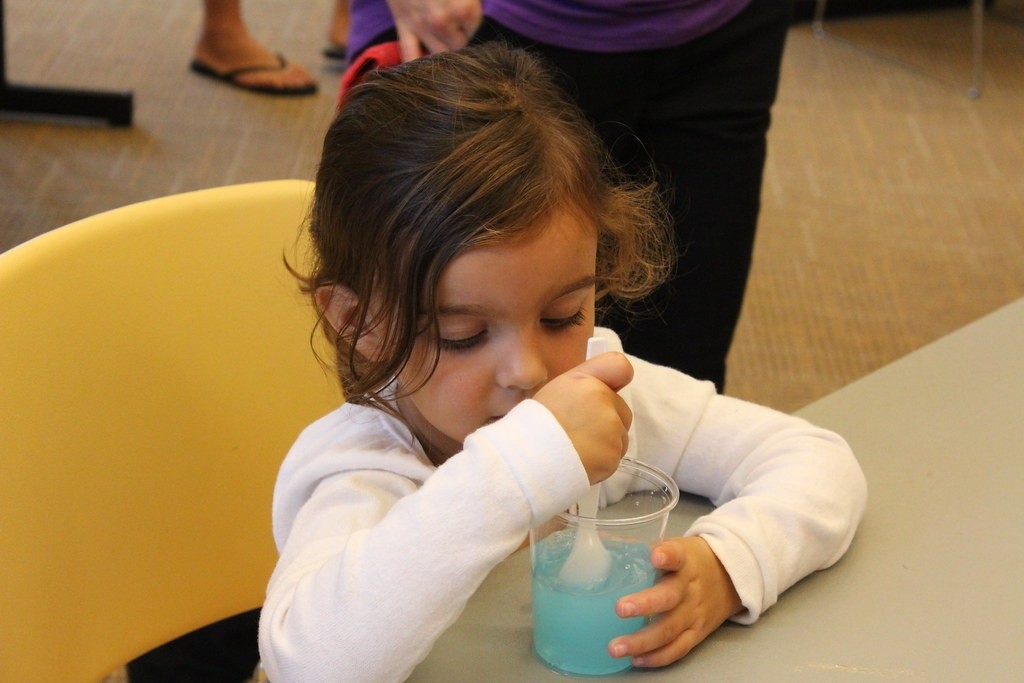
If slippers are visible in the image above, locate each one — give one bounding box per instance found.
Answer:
[191,48,318,95]
[323,46,348,59]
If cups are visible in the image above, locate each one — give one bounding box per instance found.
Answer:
[529,457,680,678]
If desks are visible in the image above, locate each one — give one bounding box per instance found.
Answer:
[406,293,1023,683]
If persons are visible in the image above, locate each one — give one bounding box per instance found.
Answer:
[187,0,350,94]
[256,41,867,682]
[349,0,791,392]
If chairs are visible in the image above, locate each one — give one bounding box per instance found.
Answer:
[0,180,351,683]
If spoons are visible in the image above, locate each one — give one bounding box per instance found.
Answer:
[555,337,615,592]
